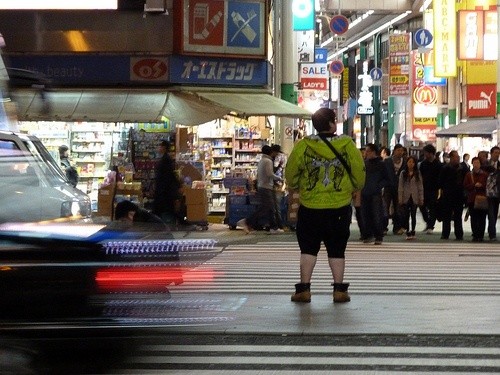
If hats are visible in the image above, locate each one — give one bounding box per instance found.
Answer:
[271,145,282,153]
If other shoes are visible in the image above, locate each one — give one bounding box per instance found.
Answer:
[426,228,433,234]
[406,231,415,240]
[374,240,381,244]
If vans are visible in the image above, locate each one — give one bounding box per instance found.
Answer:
[0,130,91,222]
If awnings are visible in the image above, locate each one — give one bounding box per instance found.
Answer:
[436,118,500,138]
[11,87,314,127]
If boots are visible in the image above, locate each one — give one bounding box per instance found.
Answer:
[332,283,350,302]
[291,283,311,303]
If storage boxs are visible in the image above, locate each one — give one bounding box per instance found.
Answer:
[97,185,113,216]
[186,188,208,204]
[187,205,207,222]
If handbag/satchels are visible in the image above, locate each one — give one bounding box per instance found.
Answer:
[473,195,488,209]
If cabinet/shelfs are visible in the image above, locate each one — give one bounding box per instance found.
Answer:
[17,120,271,223]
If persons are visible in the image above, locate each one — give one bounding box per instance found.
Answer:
[237,144,285,235]
[154,139,178,233]
[398,156,424,240]
[462,156,488,243]
[59,145,79,189]
[284,108,366,303]
[352,143,500,245]
